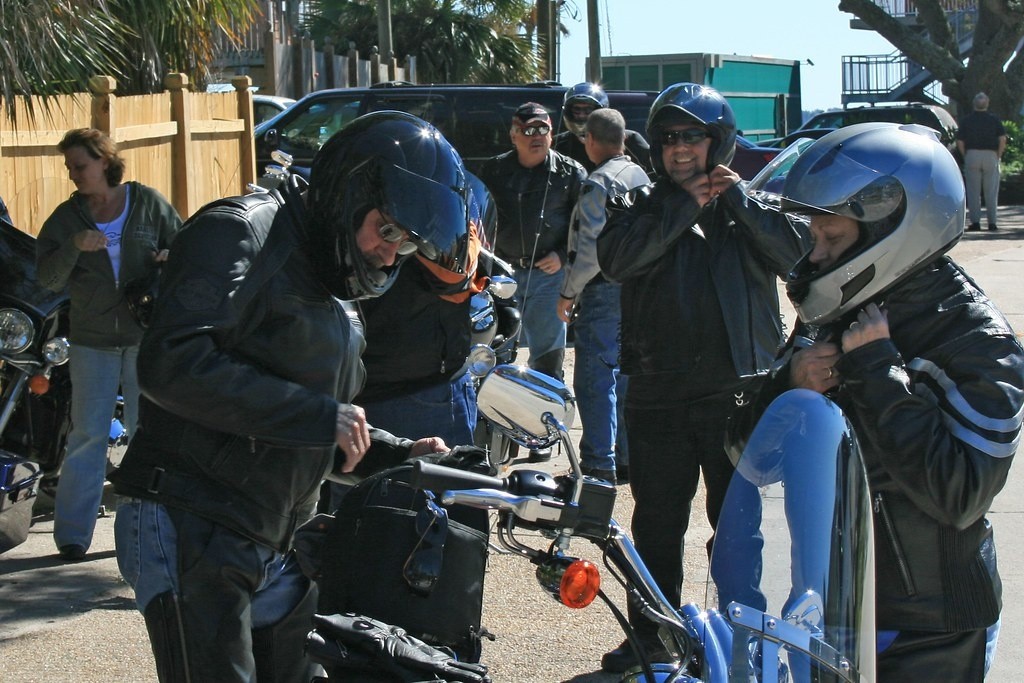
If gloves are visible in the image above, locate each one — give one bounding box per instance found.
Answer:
[304,611,492,683]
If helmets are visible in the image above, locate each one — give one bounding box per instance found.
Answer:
[562,83,608,137]
[411,230,483,297]
[646,83,737,182]
[777,124,966,329]
[305,110,468,301]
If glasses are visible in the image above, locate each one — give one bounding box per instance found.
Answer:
[570,107,595,116]
[661,129,716,146]
[522,126,549,137]
[402,502,449,595]
[374,206,417,255]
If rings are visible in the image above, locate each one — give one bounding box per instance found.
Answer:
[827,368,833,378]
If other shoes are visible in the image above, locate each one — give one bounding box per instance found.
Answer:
[968,223,980,231]
[989,223,997,230]
[60,544,84,559]
[554,464,630,485]
[601,629,675,673]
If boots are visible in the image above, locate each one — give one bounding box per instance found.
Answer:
[529,349,566,463]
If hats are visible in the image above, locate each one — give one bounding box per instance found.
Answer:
[512,102,552,131]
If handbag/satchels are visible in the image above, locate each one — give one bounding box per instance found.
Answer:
[122,268,161,331]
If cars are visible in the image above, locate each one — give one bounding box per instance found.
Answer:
[253,77,960,183]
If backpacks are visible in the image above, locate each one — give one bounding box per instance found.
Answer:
[319,440,496,660]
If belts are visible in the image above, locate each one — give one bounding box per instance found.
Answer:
[504,257,542,269]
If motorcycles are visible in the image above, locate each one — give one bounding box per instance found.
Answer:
[1,219,133,556]
[465,273,525,476]
[292,364,879,683]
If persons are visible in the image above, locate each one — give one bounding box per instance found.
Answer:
[35,127,186,556]
[303,79,660,668]
[723,123,1024,683]
[595,83,784,671]
[957,92,1006,231]
[114,109,465,683]
